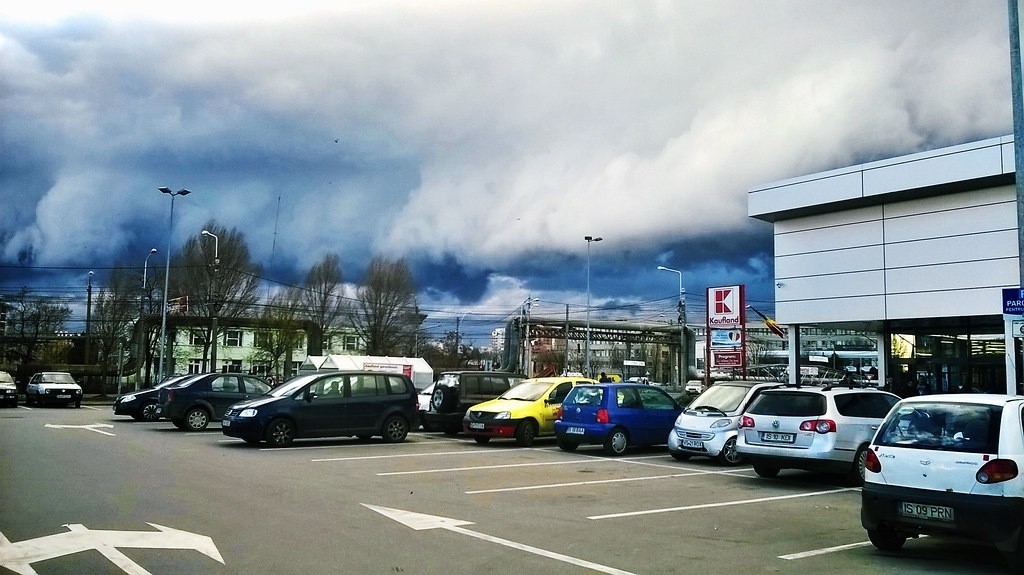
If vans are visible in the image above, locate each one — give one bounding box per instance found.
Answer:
[220,370,422,448]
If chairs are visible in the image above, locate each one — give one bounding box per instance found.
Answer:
[622,393,634,406]
[222,382,236,392]
[327,381,338,396]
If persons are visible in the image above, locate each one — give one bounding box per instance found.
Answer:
[840,372,856,384]
[599,372,612,383]
[950,381,972,392]
[637,376,649,385]
[901,376,931,398]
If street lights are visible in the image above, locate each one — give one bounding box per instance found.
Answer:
[657,265,686,395]
[457,311,471,372]
[525,298,540,378]
[134,247,158,391]
[583,235,603,379]
[156,185,192,387]
[201,229,219,373]
[82,270,94,394]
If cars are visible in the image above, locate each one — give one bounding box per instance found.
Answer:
[25,371,83,408]
[461,373,607,448]
[553,382,687,457]
[112,372,257,422]
[158,371,276,433]
[667,380,789,466]
[0,370,18,408]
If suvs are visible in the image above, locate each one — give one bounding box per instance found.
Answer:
[733,383,933,490]
[858,391,1024,575]
[422,368,531,436]
